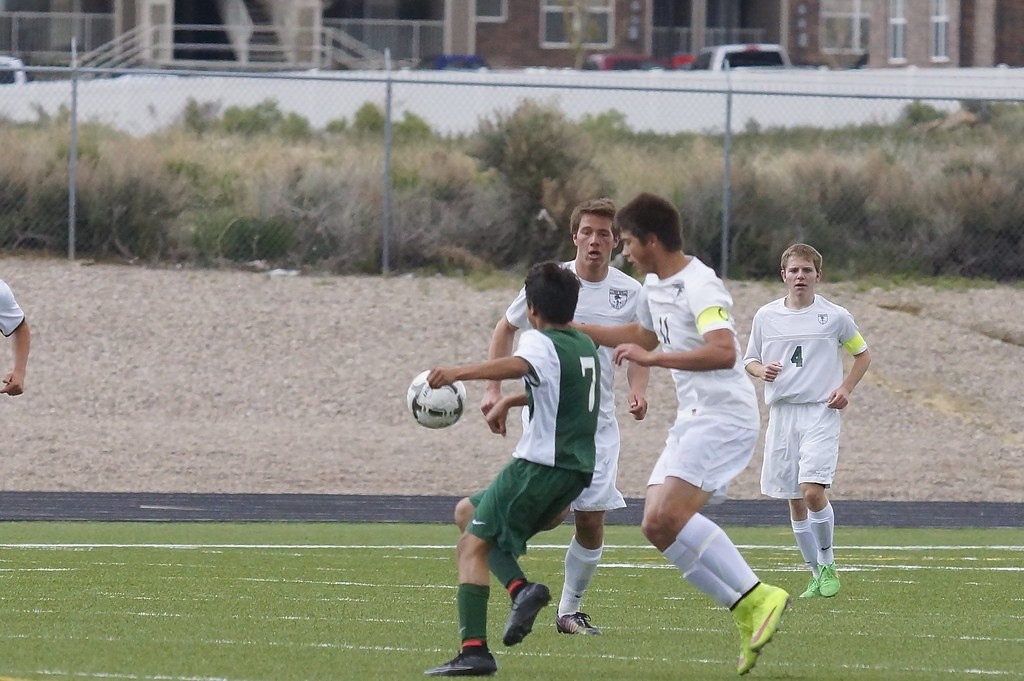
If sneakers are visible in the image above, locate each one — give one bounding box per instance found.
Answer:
[750,585,790,650]
[503,583,551,646]
[734,597,760,675]
[800,580,822,598]
[424,649,497,675]
[818,560,841,597]
[556,610,602,635]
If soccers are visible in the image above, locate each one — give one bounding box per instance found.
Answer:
[406,369,467,430]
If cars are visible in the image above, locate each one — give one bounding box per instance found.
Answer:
[409,43,794,69]
[0,55,25,84]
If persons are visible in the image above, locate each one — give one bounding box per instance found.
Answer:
[0,277,30,398]
[743,244,870,598]
[568,191,790,677]
[482,199,649,640]
[406,261,600,675]
